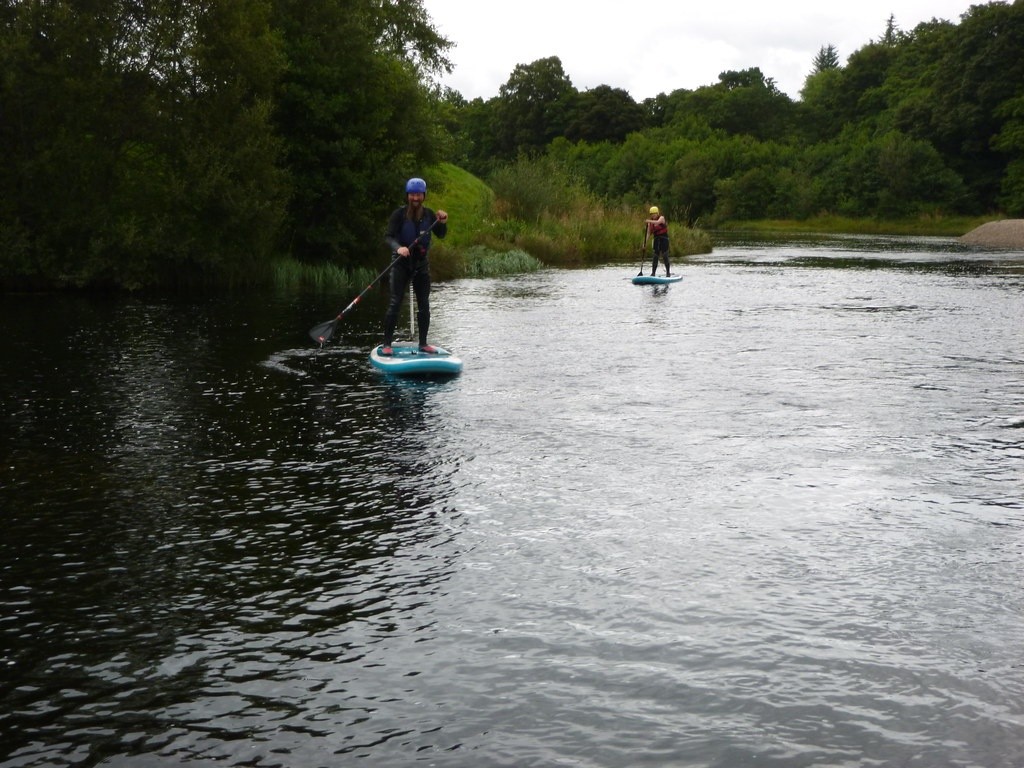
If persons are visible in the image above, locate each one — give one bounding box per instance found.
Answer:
[643,206,670,277]
[382,178,448,355]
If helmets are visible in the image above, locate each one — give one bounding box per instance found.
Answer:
[405,177,426,193]
[650,206,660,216]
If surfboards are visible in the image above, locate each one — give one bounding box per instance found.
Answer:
[632,273,683,284]
[371,342,462,375]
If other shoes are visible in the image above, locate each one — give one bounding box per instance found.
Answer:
[419,345,438,353]
[382,347,393,356]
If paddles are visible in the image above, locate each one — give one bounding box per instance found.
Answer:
[310,217,440,345]
[637,221,648,277]
